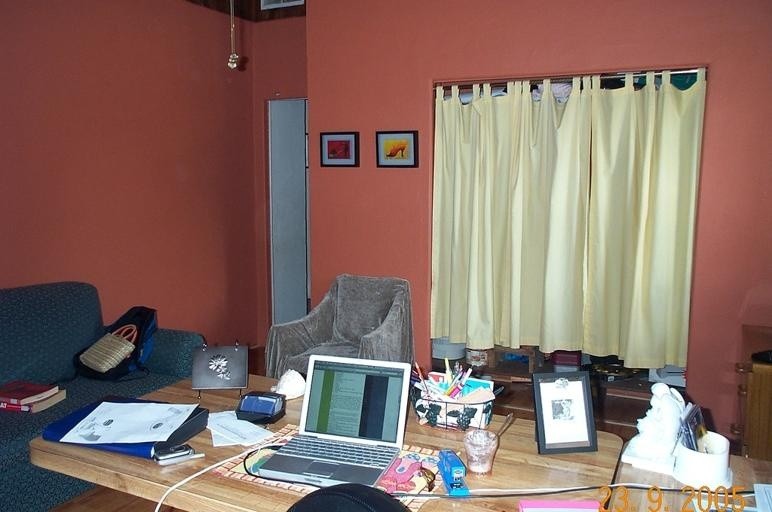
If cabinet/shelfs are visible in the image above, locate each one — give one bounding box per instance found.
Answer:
[730,350,772,461]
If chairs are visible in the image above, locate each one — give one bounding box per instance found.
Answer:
[264,274,415,379]
[286,483,412,512]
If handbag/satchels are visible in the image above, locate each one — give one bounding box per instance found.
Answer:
[72,305,158,383]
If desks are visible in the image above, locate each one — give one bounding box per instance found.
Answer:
[607,441,772,512]
[433,350,684,440]
[30,374,622,512]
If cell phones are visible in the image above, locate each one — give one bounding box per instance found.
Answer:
[153,443,206,466]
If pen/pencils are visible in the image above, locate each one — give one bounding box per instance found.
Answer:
[410,356,473,399]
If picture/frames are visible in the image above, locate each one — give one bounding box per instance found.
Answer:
[319,131,360,167]
[376,130,420,168]
[532,371,598,454]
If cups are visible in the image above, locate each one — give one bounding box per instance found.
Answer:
[463,427,500,480]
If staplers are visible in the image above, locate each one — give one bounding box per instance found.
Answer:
[436,448,469,497]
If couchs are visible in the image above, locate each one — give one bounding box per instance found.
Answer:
[0,281,207,512]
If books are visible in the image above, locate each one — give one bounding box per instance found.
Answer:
[0,390,67,413]
[0,381,60,406]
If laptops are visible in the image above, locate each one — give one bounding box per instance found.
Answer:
[258,354,412,489]
